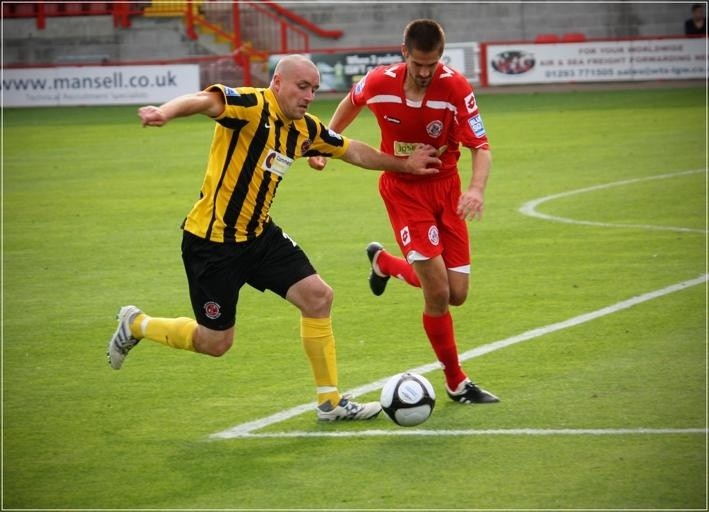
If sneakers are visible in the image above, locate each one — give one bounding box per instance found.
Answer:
[106,306,144,372]
[444,378,500,404]
[315,397,382,422]
[366,241,391,297]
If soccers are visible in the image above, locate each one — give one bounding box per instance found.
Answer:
[381,372,436,427]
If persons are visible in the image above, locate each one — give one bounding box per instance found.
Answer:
[307,19,503,405]
[685,5,705,34]
[103,51,443,422]
